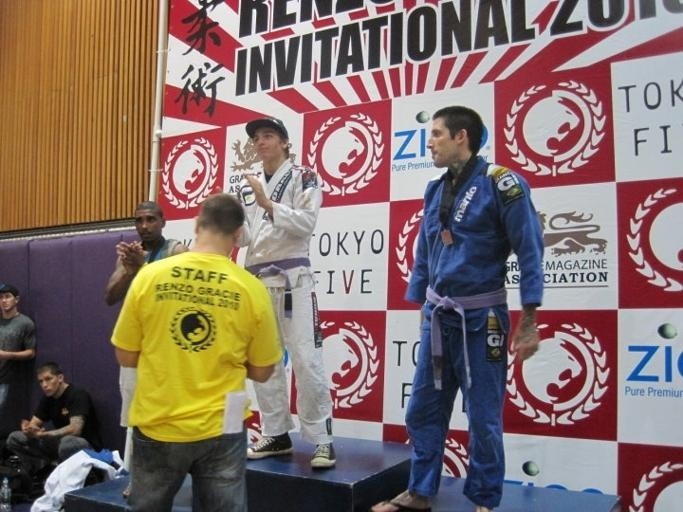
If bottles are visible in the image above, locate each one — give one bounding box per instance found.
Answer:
[0,477,12,512]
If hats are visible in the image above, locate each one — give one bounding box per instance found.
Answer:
[245,116,290,140]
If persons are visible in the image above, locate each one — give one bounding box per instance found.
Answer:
[111,195,283,512]
[0,282,38,465]
[370,105,546,512]
[6,362,101,485]
[104,200,190,505]
[230,118,339,471]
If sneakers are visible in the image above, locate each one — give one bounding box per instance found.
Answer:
[246,431,294,460]
[310,442,337,469]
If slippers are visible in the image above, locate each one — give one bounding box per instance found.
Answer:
[368,497,432,512]
